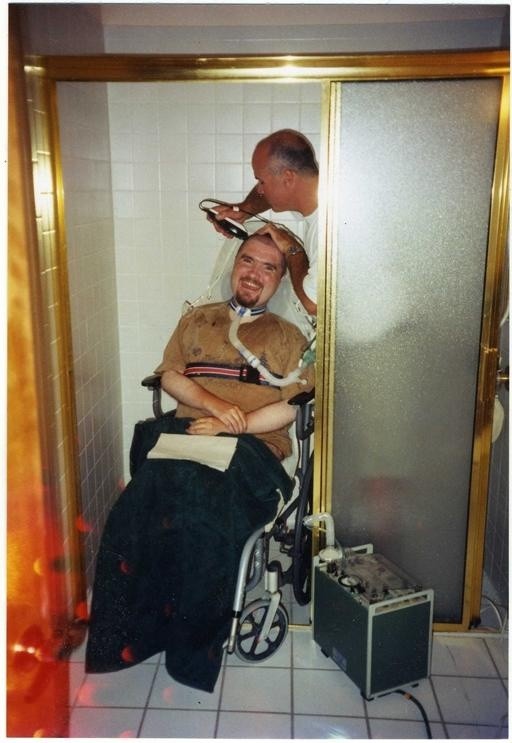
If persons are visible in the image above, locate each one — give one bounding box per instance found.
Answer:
[152,233,316,464]
[204,127,414,566]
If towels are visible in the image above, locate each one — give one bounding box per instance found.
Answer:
[147,432,239,472]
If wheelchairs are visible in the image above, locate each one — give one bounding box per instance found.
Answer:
[123,363,326,667]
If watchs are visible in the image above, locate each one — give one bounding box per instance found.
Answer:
[284,244,304,259]
